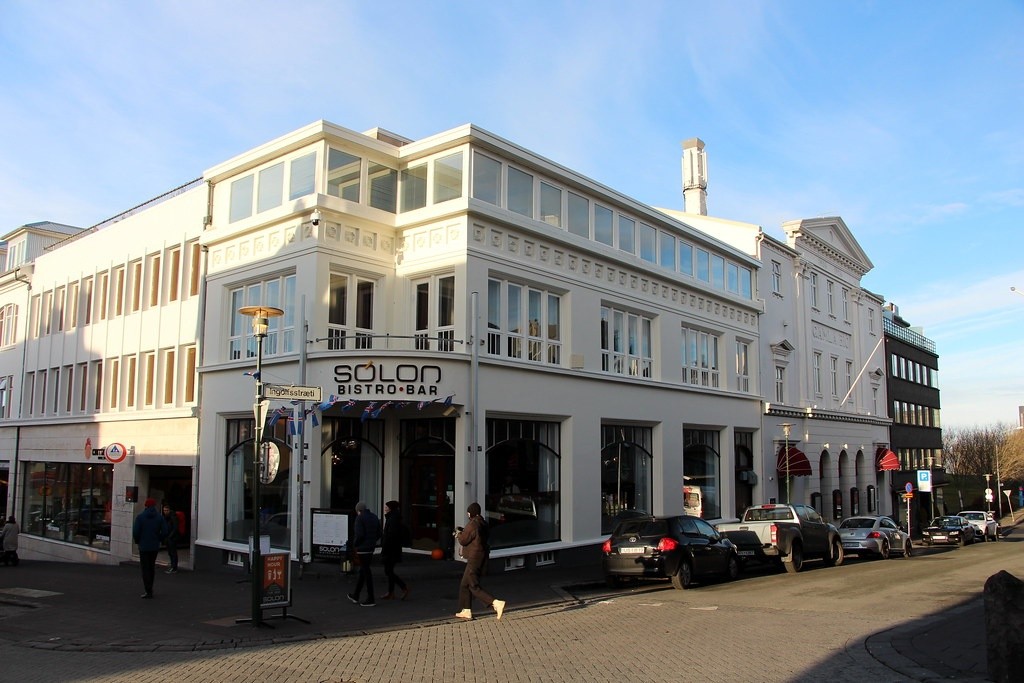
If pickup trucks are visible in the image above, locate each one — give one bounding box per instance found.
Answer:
[713,502,843,574]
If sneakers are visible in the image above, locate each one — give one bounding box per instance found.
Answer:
[361,599,376,607]
[347,594,359,603]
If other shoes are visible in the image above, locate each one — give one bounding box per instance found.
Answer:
[165,568,178,574]
[497,600,506,619]
[402,588,409,597]
[381,592,395,599]
[456,609,473,619]
[141,593,151,599]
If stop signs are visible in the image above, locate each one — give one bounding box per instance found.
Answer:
[905,482,912,493]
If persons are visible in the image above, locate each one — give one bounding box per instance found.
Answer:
[453,502,506,619]
[346,501,381,606]
[131,496,167,599]
[162,506,179,574]
[379,500,410,601]
[0,515,23,566]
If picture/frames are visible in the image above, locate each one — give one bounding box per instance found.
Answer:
[812,491,823,516]
[833,489,842,519]
[868,488,875,511]
[851,488,859,516]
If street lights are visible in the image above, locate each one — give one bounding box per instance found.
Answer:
[237,303,284,627]
[777,422,795,503]
[981,473,996,514]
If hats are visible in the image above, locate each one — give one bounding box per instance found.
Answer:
[386,501,399,511]
[468,502,480,518]
[145,497,155,506]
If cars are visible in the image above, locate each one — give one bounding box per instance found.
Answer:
[837,516,912,559]
[922,509,1000,549]
[603,515,740,590]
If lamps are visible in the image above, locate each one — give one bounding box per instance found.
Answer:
[860,446,865,449]
[844,443,848,449]
[824,444,830,449]
